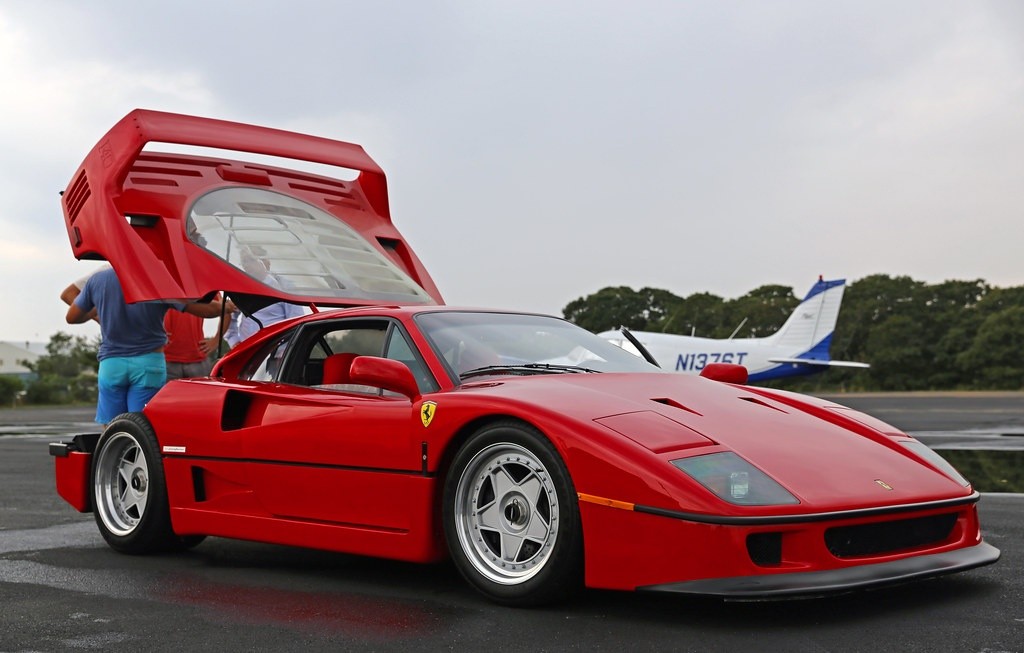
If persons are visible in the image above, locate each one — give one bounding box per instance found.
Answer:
[65,214,238,428]
[61,262,115,323]
[162,232,233,381]
[223,246,304,351]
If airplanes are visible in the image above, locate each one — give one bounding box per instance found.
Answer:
[558,278,871,387]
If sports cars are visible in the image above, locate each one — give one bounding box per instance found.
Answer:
[46,108,1000,606]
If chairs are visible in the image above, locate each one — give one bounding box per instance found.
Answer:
[323,353,367,389]
[459,351,504,384]
